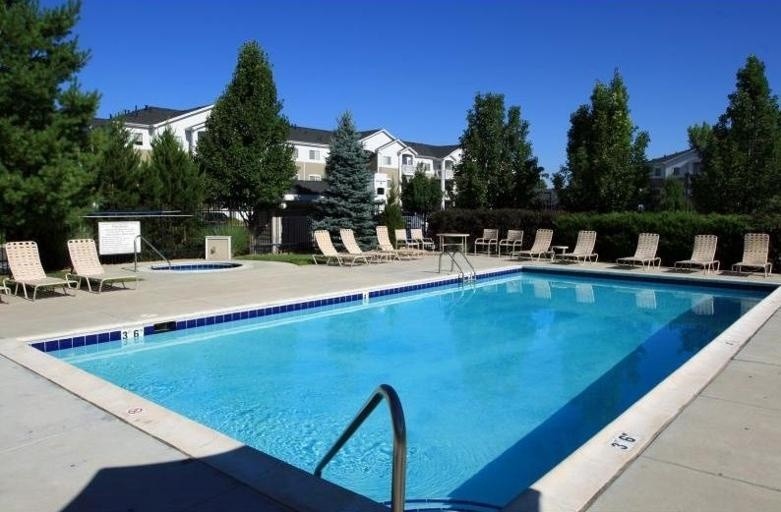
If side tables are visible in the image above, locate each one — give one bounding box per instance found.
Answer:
[435,232,472,254]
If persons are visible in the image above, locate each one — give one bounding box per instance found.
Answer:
[638,204,646,213]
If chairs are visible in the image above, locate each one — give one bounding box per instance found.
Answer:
[310,229,365,267]
[615,233,661,271]
[474,228,500,256]
[410,227,436,255]
[1,241,79,303]
[731,233,773,278]
[339,228,394,264]
[375,225,424,260]
[64,238,138,294]
[555,230,598,266]
[497,229,524,257]
[393,228,420,251]
[510,228,554,263]
[674,235,720,274]
